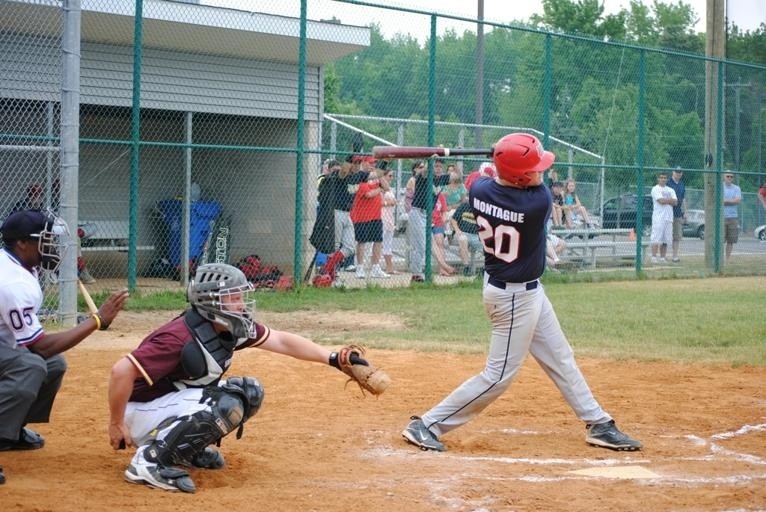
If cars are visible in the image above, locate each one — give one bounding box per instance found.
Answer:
[754,224,766,240]
[683,209,706,239]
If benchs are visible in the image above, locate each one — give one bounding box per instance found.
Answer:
[451,214,657,268]
[78,216,156,252]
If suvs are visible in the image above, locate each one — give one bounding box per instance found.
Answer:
[577,195,654,240]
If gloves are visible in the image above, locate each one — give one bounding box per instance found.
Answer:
[329,350,368,370]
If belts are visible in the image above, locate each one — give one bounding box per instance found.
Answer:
[488,278,539,291]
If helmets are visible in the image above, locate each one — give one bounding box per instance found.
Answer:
[493,132,556,188]
[1,211,70,284]
[186,261,257,340]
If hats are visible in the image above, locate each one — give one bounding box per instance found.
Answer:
[363,155,379,163]
[352,155,363,162]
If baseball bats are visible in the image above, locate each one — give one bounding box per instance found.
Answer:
[373,147,493,158]
[303,226,329,286]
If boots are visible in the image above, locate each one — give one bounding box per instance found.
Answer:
[323,250,345,280]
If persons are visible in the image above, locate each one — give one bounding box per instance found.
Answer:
[309,153,591,283]
[8,176,95,286]
[400,132,644,453]
[723,170,742,265]
[109,262,394,493]
[757,183,766,210]
[666,166,688,262]
[0,209,129,451]
[650,174,678,263]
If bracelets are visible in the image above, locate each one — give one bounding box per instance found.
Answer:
[92,313,102,331]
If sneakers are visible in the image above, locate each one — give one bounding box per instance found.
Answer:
[79,271,96,284]
[0,427,44,451]
[371,267,391,278]
[124,454,179,491]
[403,420,445,450]
[585,420,643,450]
[355,268,365,279]
[344,264,357,272]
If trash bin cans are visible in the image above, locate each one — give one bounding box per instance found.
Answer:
[157,200,222,265]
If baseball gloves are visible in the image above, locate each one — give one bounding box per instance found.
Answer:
[339,345,390,397]
